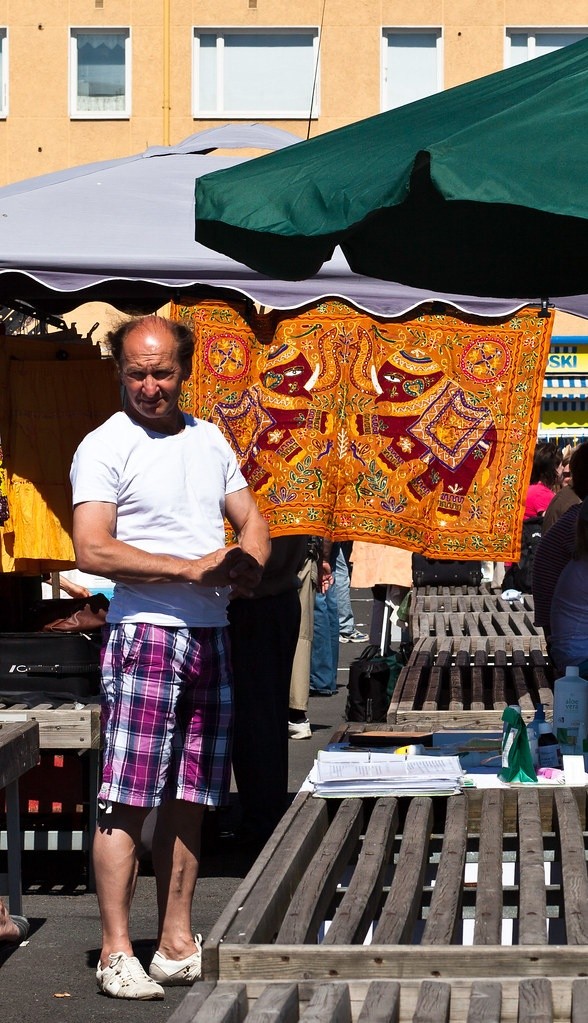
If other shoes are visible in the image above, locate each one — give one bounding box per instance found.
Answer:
[289,718,313,739]
[0,915,29,953]
[218,830,271,846]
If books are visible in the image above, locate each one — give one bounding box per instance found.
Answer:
[312,749,464,799]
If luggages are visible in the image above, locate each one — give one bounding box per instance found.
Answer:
[412,552,482,587]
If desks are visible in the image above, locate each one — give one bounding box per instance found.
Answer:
[0,720,41,916]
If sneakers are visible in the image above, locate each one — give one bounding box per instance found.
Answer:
[149,934,203,986]
[96,952,166,1001]
[338,628,370,644]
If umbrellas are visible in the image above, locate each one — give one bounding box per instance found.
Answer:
[0,123,588,322]
[194,34,588,300]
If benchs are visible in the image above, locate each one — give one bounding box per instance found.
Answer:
[167,585,588,1023]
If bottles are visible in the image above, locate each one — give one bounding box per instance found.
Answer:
[538,723,561,767]
[553,666,588,766]
[527,728,538,767]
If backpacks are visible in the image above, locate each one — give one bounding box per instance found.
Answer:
[371,653,404,701]
[346,644,391,724]
[501,510,545,595]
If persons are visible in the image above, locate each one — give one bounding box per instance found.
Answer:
[68,315,271,998]
[495,439,588,691]
[44,574,91,599]
[201,534,494,863]
